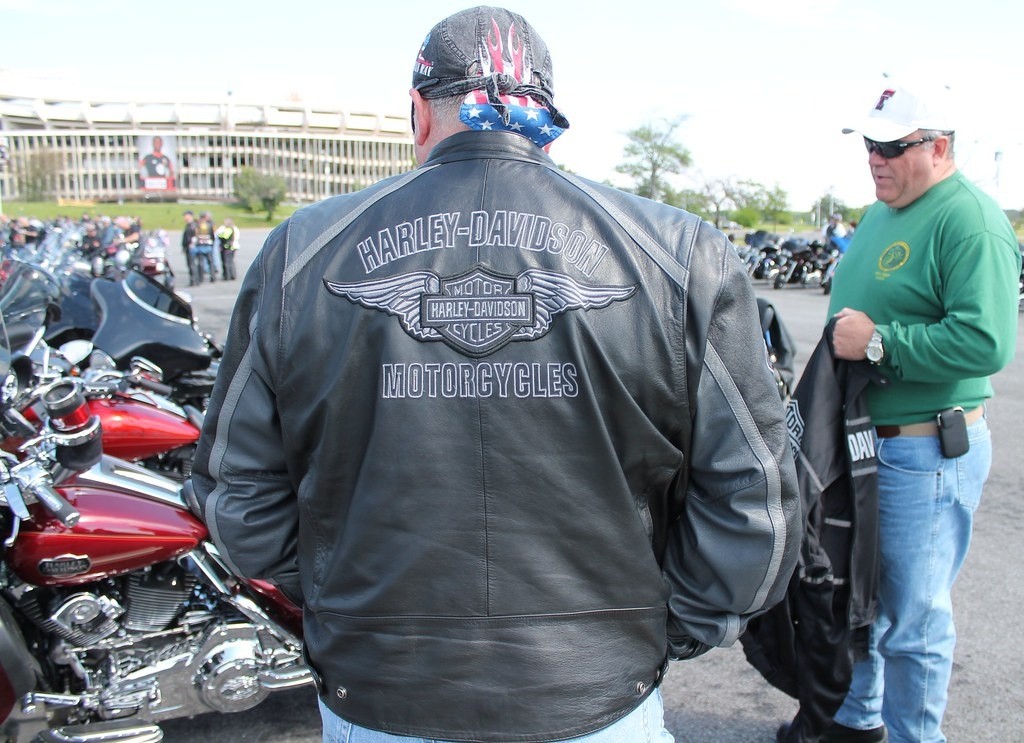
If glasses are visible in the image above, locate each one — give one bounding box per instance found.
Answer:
[864,131,954,159]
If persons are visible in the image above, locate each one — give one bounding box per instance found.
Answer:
[188,4,803,743]
[822,215,858,294]
[775,83,1022,743]
[0,213,143,280]
[183,211,240,286]
[140,137,174,177]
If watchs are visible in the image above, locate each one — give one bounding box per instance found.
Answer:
[864,331,884,366]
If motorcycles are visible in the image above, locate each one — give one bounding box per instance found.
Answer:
[0,223,321,743]
[734,226,1023,311]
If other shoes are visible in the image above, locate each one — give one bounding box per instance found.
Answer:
[776,714,884,743]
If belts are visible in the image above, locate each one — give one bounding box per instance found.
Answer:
[874,406,984,438]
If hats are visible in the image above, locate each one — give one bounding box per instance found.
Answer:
[831,214,842,221]
[842,85,957,142]
